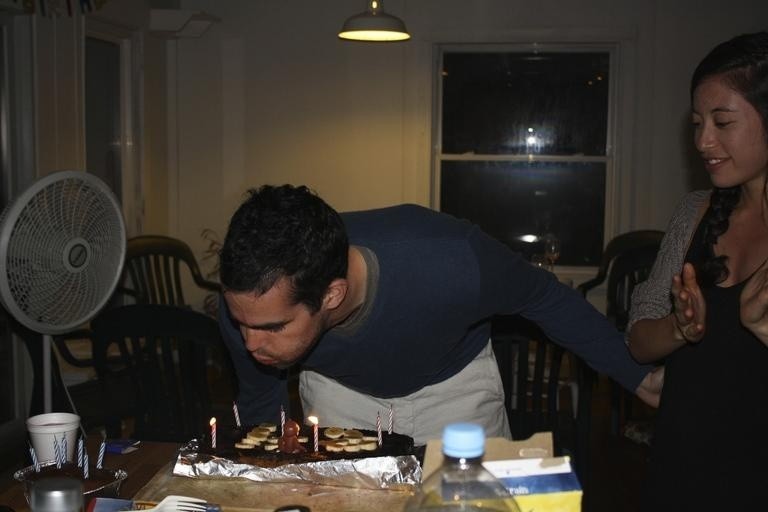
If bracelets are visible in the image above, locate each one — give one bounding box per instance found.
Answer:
[13,464,128,511]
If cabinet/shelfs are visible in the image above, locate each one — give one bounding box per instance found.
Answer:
[403,423,521,512]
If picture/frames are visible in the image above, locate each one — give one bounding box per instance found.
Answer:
[62,435,586,511]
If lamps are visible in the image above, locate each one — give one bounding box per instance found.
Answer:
[92,304,240,445]
[88,237,222,409]
[491,313,593,453]
[572,230,668,397]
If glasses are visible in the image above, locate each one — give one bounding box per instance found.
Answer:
[338,1,413,43]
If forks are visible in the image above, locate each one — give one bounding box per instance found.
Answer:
[123,495,207,512]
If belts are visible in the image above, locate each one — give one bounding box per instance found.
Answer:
[543,239,561,272]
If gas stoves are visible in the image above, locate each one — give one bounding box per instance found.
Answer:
[179,423,415,469]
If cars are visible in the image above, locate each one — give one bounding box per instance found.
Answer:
[26,412,81,463]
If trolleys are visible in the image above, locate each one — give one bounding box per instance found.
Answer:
[0,169,131,411]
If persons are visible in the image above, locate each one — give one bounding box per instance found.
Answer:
[218,181,665,436]
[625,23,767,508]
